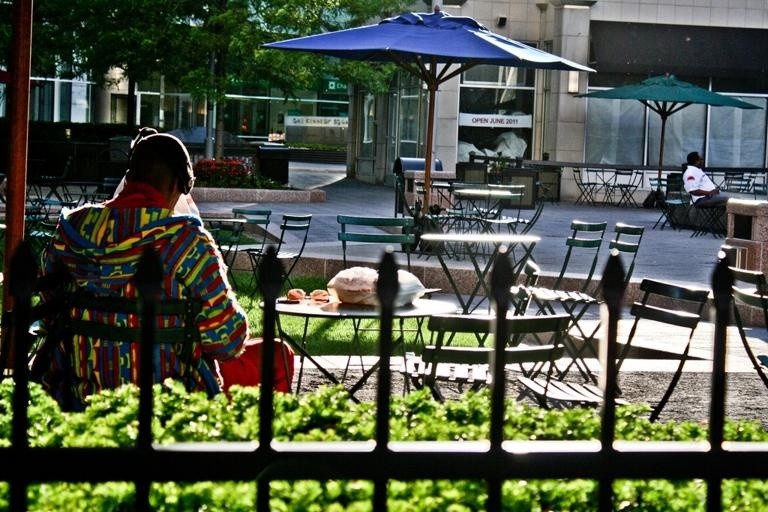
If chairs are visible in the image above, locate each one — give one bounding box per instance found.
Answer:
[0,131,767,422]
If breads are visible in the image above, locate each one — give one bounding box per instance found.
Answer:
[289,288,305,299]
[311,289,329,301]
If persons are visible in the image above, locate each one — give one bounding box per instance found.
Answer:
[679,147,735,207]
[41,133,294,399]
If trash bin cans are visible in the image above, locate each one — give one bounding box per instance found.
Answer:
[393,156,442,219]
[258,146,288,185]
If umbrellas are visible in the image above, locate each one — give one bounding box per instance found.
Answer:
[257,5,597,246]
[570,73,766,192]
